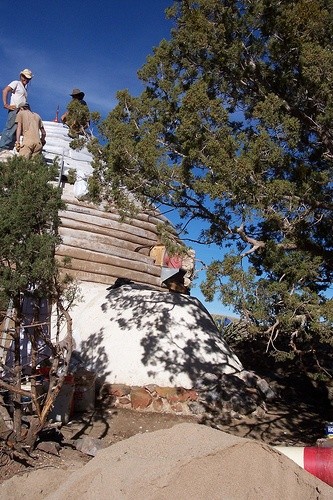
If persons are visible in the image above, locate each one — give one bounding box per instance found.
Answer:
[61,89,90,135]
[0,68,34,151]
[15,102,46,164]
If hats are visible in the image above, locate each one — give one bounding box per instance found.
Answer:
[16,102,30,113]
[70,89,85,100]
[19,69,33,79]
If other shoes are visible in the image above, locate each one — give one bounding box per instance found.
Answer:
[68,132,76,138]
